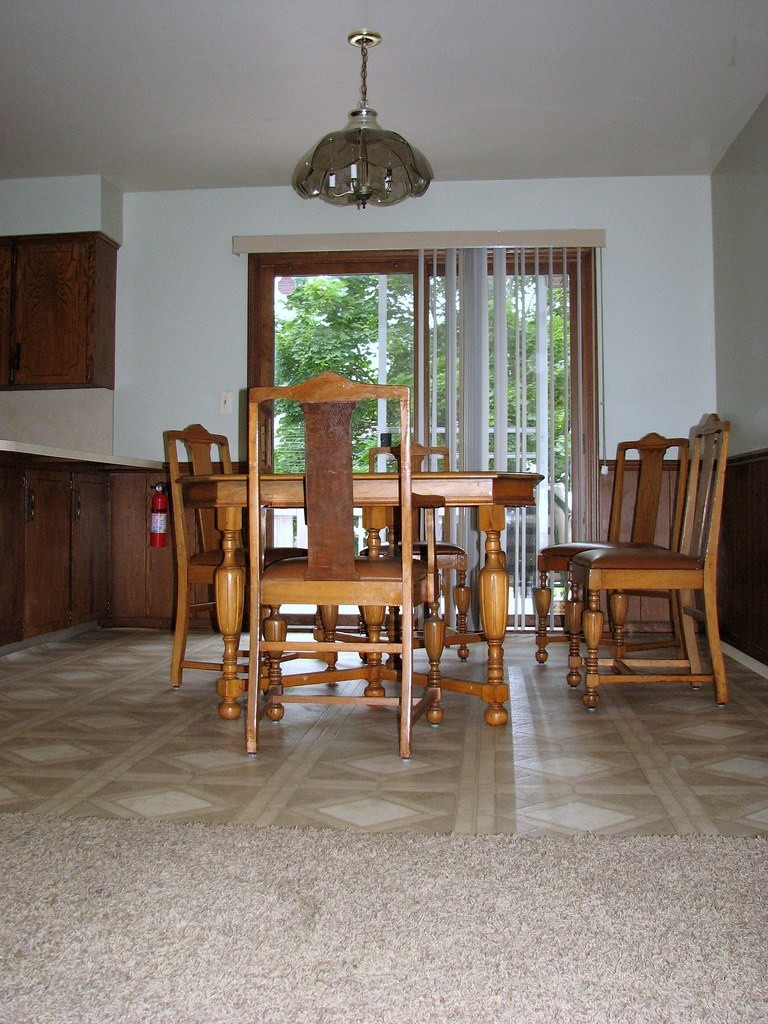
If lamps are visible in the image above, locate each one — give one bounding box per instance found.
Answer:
[290,30,434,210]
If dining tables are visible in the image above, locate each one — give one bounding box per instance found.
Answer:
[175,470,544,727]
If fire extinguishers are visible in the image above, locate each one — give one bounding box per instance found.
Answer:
[148,479,169,548]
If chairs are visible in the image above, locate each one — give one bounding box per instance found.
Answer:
[359,444,468,662]
[533,432,689,675]
[246,370,445,764]
[163,424,339,724]
[566,413,731,710]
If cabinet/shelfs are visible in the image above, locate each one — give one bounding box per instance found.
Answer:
[0,230,121,391]
[109,463,252,632]
[0,464,108,652]
